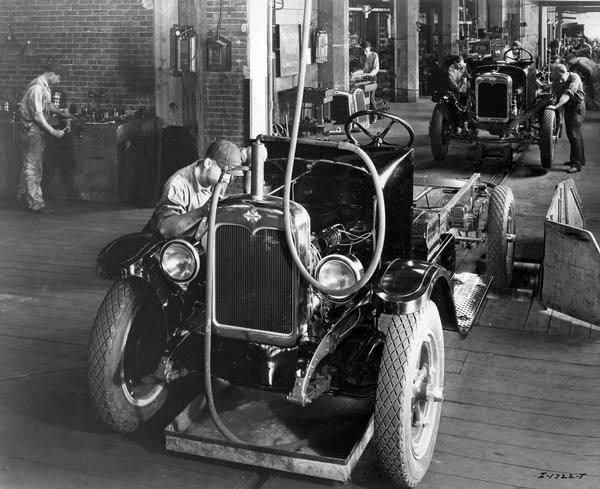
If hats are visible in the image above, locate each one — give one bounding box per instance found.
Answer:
[46,63,64,76]
[551,64,567,80]
[205,140,244,177]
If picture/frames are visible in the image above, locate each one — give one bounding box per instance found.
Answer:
[277,24,302,78]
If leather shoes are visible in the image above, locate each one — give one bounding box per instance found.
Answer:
[36,206,55,214]
[567,167,578,172]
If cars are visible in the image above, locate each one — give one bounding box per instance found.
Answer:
[89,110,517,488]
[430,47,560,169]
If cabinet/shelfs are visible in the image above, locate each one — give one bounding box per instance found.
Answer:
[0,121,139,205]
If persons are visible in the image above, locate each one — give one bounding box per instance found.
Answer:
[447,55,472,133]
[17,64,68,214]
[360,40,380,124]
[143,139,269,239]
[545,63,587,173]
[499,40,523,62]
[564,53,600,110]
[571,38,592,59]
